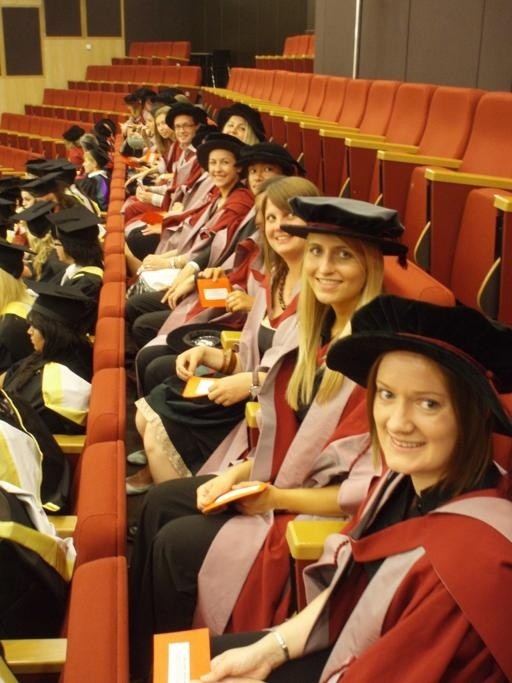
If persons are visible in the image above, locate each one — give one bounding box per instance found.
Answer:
[201,296,512,683]
[64,117,117,213]
[0,157,106,683]
[120,88,319,493]
[127,196,408,682]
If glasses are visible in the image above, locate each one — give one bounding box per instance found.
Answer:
[175,123,198,130]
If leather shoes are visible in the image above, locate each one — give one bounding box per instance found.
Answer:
[125,448,152,497]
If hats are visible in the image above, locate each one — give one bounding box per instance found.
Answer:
[80,140,113,167]
[63,122,84,141]
[95,118,116,135]
[150,371,246,425]
[278,195,412,271]
[0,158,106,319]
[323,293,510,435]
[122,86,306,174]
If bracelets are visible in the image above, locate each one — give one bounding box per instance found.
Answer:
[274,630,291,661]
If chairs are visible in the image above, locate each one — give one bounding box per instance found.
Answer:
[1,40,201,176]
[219,253,512,614]
[199,36,512,335]
[1,129,132,683]
